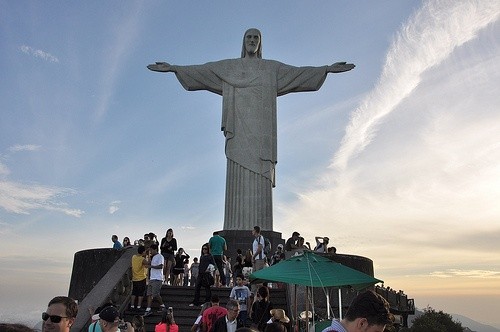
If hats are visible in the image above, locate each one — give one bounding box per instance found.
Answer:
[92,306,120,323]
[270,309,289,323]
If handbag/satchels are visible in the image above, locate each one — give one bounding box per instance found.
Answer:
[249,323,259,331]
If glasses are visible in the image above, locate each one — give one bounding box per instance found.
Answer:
[123,239,128,242]
[202,248,208,250]
[42,312,71,324]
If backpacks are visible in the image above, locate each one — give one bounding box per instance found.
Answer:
[256,235,271,256]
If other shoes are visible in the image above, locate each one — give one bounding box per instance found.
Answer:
[136,305,141,308]
[160,304,165,308]
[189,304,194,306]
[201,303,204,306]
[131,305,134,308]
[145,308,152,312]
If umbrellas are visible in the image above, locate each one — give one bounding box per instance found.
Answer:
[248,249,384,332]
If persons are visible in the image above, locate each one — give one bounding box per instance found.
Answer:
[322,289,396,332]
[146,28,356,231]
[41,296,79,332]
[88,225,337,332]
